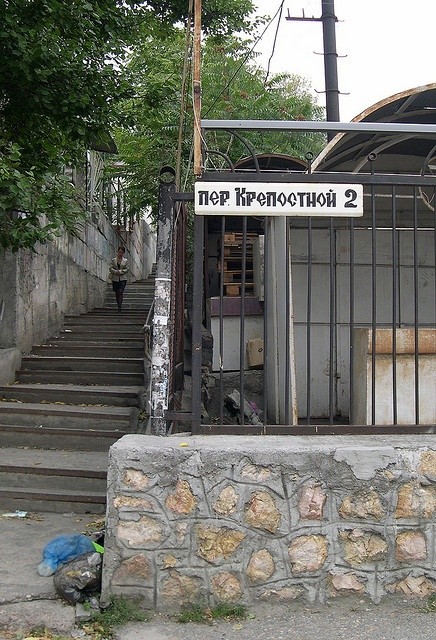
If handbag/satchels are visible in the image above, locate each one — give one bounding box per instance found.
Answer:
[108,272,112,280]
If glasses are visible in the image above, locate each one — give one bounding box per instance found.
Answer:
[118,251,123,253]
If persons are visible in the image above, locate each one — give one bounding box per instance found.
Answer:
[108,246,129,312]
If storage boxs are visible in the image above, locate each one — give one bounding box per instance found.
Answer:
[247,338,264,367]
